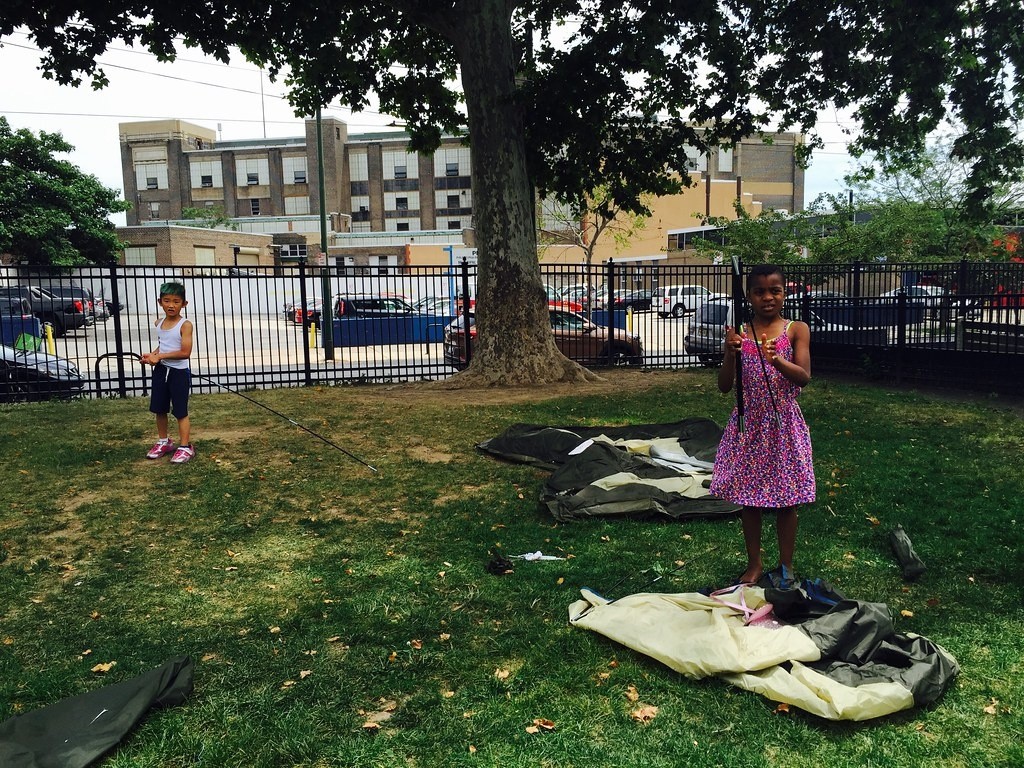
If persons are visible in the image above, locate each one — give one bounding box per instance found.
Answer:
[715,264,811,591]
[139,283,196,463]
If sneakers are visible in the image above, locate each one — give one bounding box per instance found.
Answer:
[170,443,196,463]
[147,438,174,459]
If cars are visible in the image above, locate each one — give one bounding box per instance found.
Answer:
[444,305,644,370]
[684,290,874,366]
[414,285,653,317]
[876,285,983,320]
[284,298,324,328]
[0,284,126,337]
[0,343,87,401]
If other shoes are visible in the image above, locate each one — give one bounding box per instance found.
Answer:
[733,569,746,585]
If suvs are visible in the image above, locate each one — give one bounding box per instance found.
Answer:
[651,285,731,318]
[331,292,418,317]
